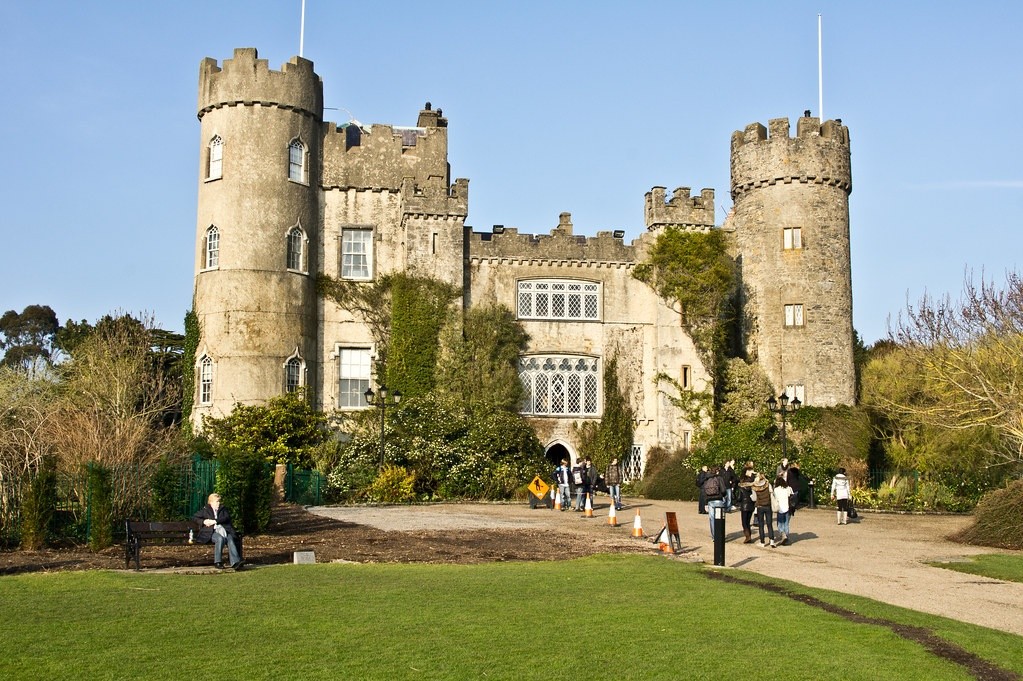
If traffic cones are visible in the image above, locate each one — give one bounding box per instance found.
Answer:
[628,509,647,540]
[551,488,565,511]
[580,492,597,518]
[658,519,676,555]
[604,497,620,527]
[550,485,556,508]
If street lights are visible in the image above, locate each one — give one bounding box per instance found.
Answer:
[766,393,801,458]
[364,384,403,467]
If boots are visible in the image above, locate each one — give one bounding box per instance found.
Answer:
[837,511,842,524]
[743,528,751,542]
[841,512,847,524]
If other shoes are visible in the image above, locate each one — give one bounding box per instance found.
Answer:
[780,534,788,545]
[698,510,709,514]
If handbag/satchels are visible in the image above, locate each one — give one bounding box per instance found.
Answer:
[770,492,779,512]
[788,494,795,516]
[750,490,757,501]
[574,473,583,484]
[847,500,857,518]
[550,471,558,481]
[753,514,760,526]
[214,524,227,537]
[556,466,563,484]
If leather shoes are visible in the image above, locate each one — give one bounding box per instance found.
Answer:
[215,562,225,568]
[234,561,245,570]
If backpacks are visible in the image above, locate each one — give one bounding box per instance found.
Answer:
[703,476,720,495]
[696,472,704,487]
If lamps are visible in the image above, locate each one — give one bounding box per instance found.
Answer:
[713,506,726,520]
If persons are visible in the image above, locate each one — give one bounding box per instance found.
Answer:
[786,460,808,510]
[717,457,737,513]
[696,465,720,514]
[551,458,575,512]
[776,458,789,483]
[194,492,246,572]
[572,455,598,513]
[703,466,728,543]
[605,456,623,511]
[830,468,852,525]
[738,471,777,548]
[737,474,756,544]
[740,459,755,483]
[772,477,795,545]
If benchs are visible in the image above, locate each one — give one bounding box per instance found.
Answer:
[124,521,243,571]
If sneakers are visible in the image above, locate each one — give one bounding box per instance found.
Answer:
[756,542,764,547]
[770,540,776,548]
